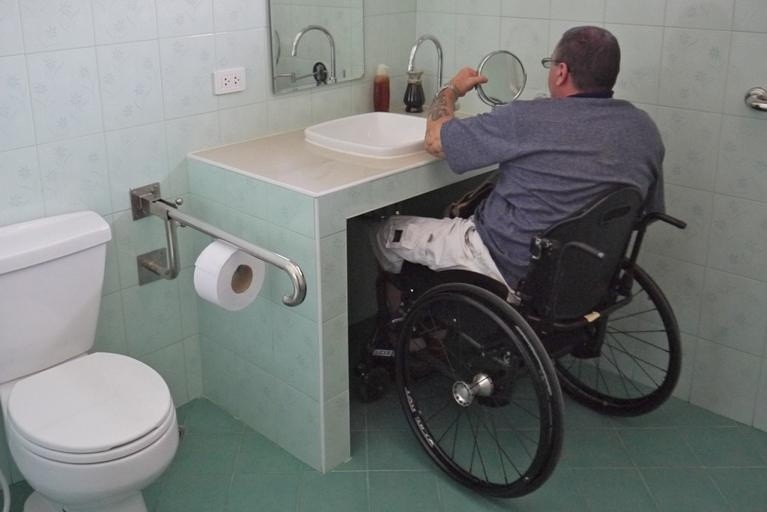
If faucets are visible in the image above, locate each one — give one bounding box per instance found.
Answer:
[291,23,338,83]
[406,33,443,93]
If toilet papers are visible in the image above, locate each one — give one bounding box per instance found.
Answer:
[192,238,267,313]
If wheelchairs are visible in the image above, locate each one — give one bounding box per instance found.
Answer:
[358,185,689,500]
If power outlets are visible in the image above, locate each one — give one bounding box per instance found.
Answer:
[209,66,247,95]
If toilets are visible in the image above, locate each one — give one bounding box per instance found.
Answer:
[0,211,181,512]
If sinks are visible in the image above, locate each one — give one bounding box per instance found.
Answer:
[309,112,427,148]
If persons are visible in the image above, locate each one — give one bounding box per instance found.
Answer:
[358,25,667,368]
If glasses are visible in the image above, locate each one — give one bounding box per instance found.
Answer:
[541,57,560,68]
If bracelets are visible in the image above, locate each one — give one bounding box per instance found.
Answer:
[434,82,463,100]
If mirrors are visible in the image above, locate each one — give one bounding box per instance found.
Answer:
[268,0,366,97]
[472,49,529,113]
